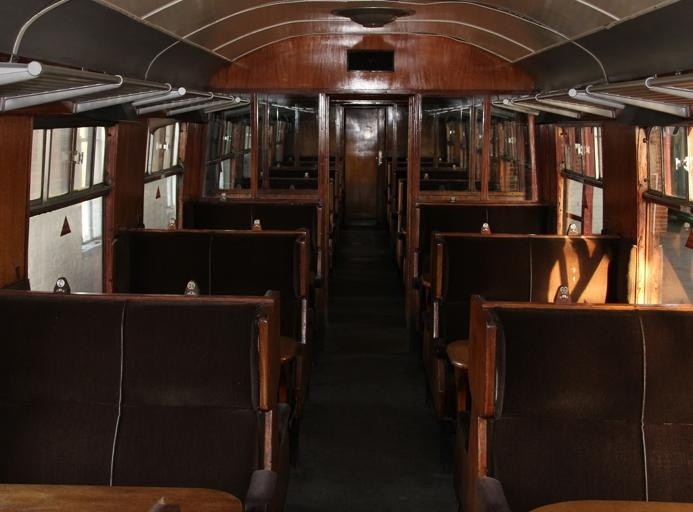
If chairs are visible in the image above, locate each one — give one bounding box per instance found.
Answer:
[2,155,340,512]
[379,157,691,511]
